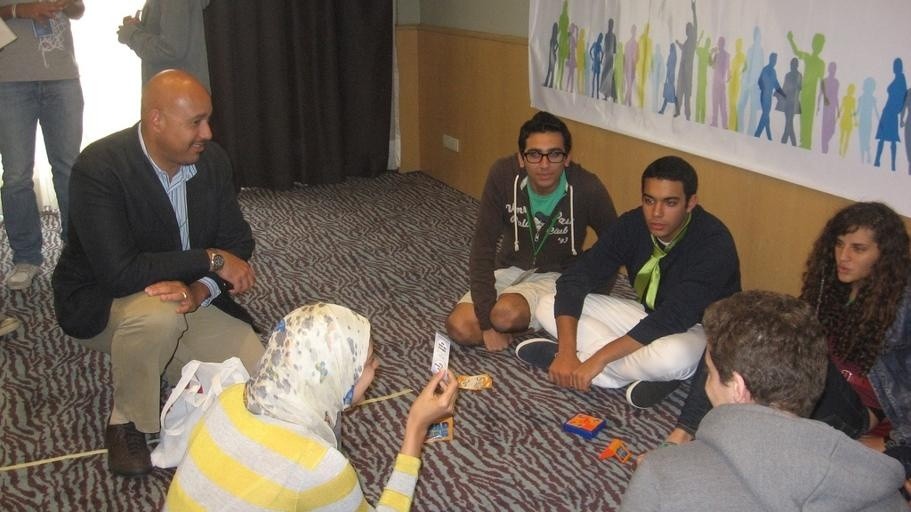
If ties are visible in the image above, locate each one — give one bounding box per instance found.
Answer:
[633,245,667,310]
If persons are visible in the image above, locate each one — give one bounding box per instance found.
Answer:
[118,1,213,115]
[161,301,460,511]
[639,204,911,472]
[446,112,617,352]
[620,288,910,510]
[513,156,741,410]
[1,1,89,292]
[57,70,277,478]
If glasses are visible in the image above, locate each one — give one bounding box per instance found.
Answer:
[522,148,567,163]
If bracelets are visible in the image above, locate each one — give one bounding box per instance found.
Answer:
[12,3,16,20]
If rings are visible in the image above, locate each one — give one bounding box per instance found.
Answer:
[183,290,189,299]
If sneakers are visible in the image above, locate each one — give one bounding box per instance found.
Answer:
[627,380,683,409]
[104,413,153,475]
[514,338,559,368]
[1,316,22,336]
[8,263,43,290]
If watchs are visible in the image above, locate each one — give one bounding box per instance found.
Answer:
[206,247,225,274]
